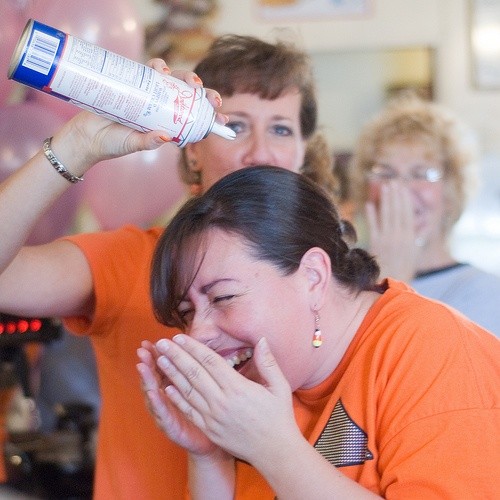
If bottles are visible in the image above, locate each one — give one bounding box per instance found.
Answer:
[6,17,237,150]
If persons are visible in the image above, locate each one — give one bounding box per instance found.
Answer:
[136,166,500,500]
[0,33,341,500]
[357,93,500,340]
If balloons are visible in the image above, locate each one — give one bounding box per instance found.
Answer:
[0,0,188,249]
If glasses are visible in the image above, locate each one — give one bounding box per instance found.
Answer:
[363,162,448,186]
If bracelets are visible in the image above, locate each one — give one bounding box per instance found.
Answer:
[42,137,83,184]
